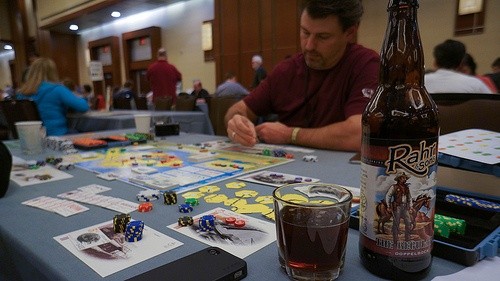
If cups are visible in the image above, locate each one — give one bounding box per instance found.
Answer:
[15,121,42,155]
[272,182,353,281]
[133,114,150,134]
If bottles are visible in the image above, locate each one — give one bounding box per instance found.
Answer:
[358,0,441,281]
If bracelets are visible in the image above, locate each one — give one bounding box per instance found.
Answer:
[292,127,299,144]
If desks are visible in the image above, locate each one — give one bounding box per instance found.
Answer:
[0,127,500,281]
[66,107,217,134]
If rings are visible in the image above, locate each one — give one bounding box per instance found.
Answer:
[232,132,235,136]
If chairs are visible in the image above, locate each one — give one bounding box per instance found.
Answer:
[91,96,244,136]
[429,91,500,134]
[0,100,42,140]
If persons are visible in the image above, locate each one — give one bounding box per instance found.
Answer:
[422,40,493,93]
[23,54,40,79]
[216,71,248,96]
[459,54,496,94]
[15,57,89,136]
[224,0,384,150]
[252,55,268,84]
[145,49,182,111]
[82,85,94,99]
[191,80,208,96]
[483,57,500,92]
[117,80,137,110]
